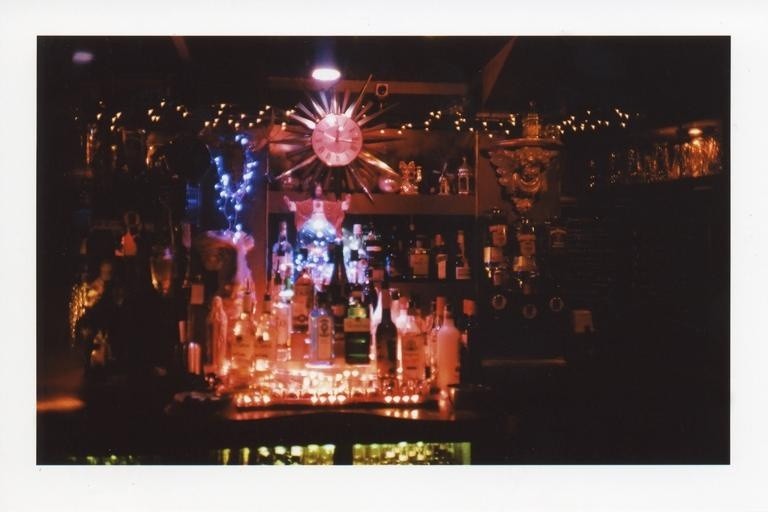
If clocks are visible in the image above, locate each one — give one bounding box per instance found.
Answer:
[272,77,406,204]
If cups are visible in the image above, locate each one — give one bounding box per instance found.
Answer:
[350,442,469,467]
[582,135,720,192]
[212,444,335,467]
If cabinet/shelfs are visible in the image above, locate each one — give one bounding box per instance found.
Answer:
[270,115,482,301]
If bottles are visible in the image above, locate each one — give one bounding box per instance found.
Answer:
[277,152,471,195]
[478,204,541,294]
[184,277,458,391]
[272,198,473,284]
